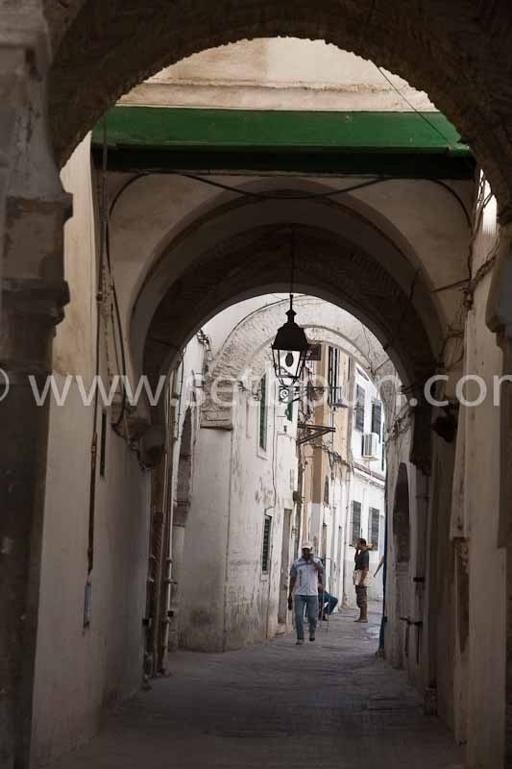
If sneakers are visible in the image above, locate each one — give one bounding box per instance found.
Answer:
[296,640,303,645]
[310,632,315,640]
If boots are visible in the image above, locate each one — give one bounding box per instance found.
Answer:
[355,605,368,623]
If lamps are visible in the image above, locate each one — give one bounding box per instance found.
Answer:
[271,292,308,380]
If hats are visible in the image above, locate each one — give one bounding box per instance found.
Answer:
[302,543,312,549]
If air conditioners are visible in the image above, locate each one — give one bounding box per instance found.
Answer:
[363,432,379,459]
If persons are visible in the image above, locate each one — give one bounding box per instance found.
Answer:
[288,541,324,644]
[315,557,338,621]
[353,538,368,623]
